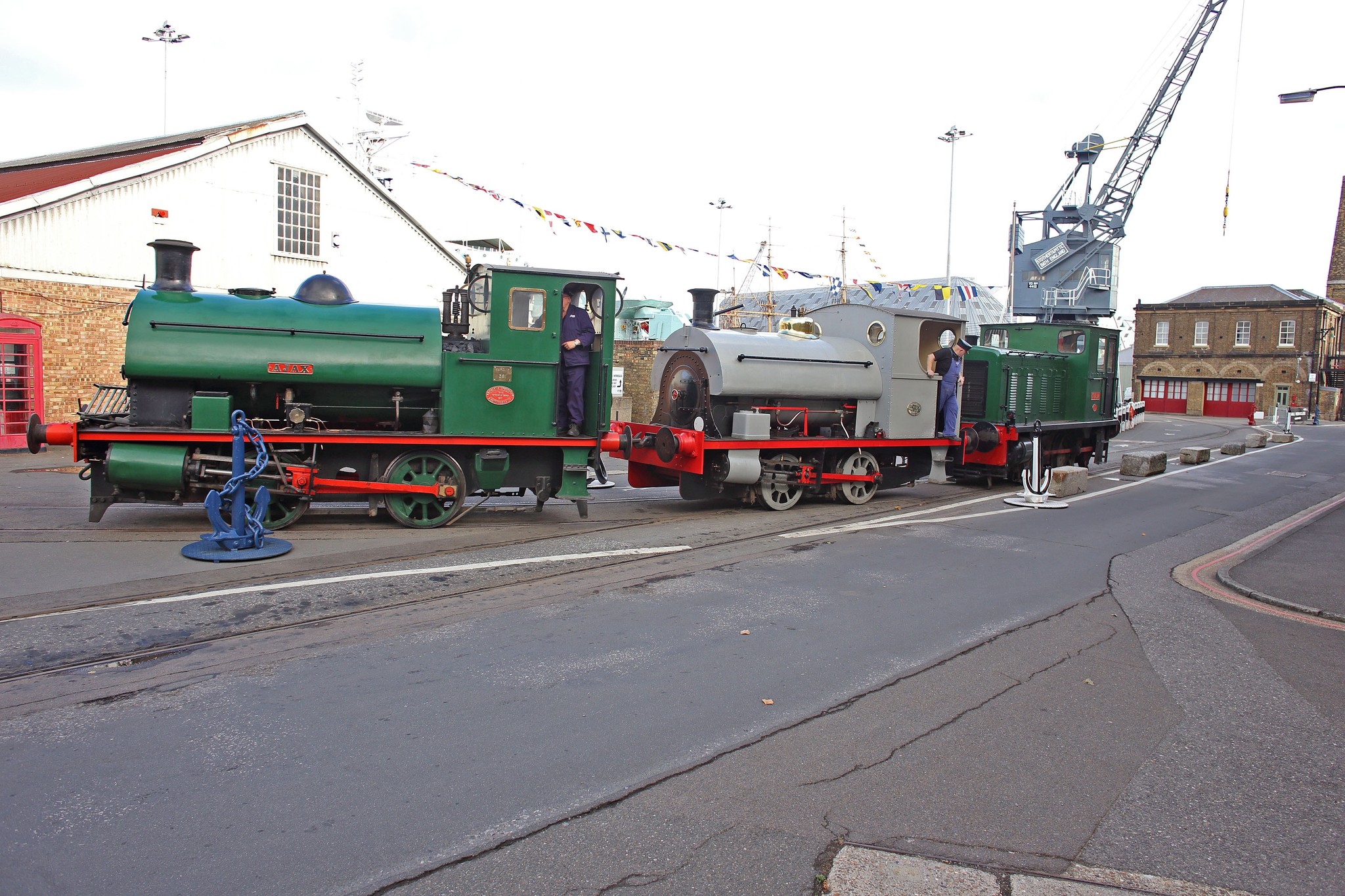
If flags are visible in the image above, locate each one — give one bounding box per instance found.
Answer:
[410,163,1002,302]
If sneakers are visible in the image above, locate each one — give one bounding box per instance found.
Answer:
[567,423,580,437]
[556,427,569,434]
[943,436,962,441]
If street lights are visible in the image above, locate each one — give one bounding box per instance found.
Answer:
[709,197,733,291]
[937,123,976,290]
[1315,325,1336,408]
[141,19,192,137]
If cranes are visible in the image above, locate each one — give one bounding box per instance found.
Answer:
[1007,1,1235,318]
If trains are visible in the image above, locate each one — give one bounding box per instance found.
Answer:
[24,202,1237,536]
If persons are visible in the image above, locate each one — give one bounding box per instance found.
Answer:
[530,289,595,437]
[927,338,972,442]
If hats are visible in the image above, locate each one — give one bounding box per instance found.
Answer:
[563,285,574,297]
[957,338,972,355]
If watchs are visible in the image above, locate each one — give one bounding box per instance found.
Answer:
[574,340,579,347]
